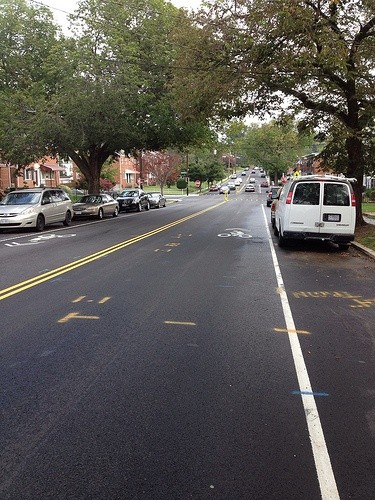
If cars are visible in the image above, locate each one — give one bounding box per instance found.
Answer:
[0,186,74,232]
[72,194,120,220]
[266,186,281,207]
[209,166,269,194]
[115,189,150,212]
[147,192,167,208]
[270,187,283,228]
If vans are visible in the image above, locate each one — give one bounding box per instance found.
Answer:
[275,173,356,250]
